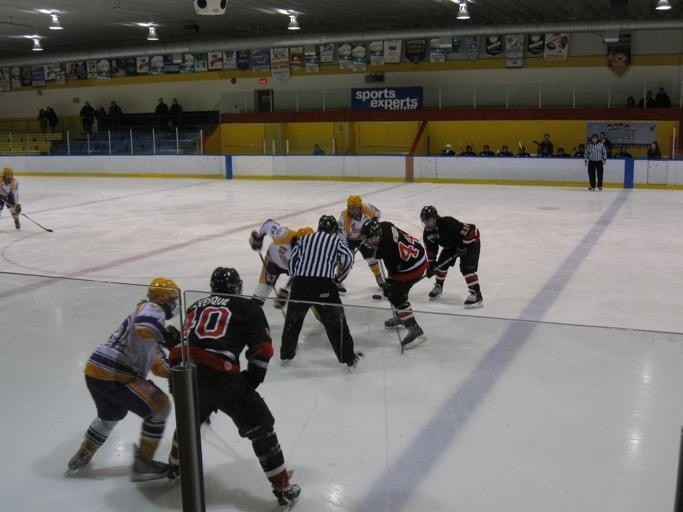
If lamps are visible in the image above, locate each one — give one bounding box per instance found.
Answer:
[29,35,43,52]
[653,0,673,9]
[455,1,470,19]
[286,8,300,31]
[47,11,62,29]
[144,25,160,41]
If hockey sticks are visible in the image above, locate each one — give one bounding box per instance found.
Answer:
[373,254,461,300]
[0,197,54,233]
[378,259,404,356]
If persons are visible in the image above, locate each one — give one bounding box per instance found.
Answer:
[625,95,637,108]
[278,215,364,367]
[67,276,183,474]
[637,90,654,110]
[248,218,323,322]
[164,266,303,505]
[1,166,23,229]
[312,144,325,155]
[418,204,484,306]
[360,217,429,346]
[583,133,607,190]
[438,131,668,158]
[653,87,671,108]
[35,96,184,140]
[333,193,387,298]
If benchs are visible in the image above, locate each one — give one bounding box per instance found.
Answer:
[0,108,224,157]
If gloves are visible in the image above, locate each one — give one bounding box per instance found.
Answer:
[249,230,264,250]
[241,370,260,388]
[274,289,288,308]
[425,262,436,278]
[454,242,466,257]
[14,204,21,214]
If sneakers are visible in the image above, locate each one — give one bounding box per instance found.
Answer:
[279,350,295,359]
[336,282,346,292]
[429,284,442,297]
[464,291,482,304]
[402,327,423,344]
[69,452,90,470]
[15,220,20,228]
[599,186,601,190]
[168,458,180,479]
[133,456,167,474]
[588,186,594,190]
[275,484,300,505]
[377,280,386,287]
[348,351,358,365]
[385,316,401,326]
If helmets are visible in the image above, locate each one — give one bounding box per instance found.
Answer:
[149,277,181,318]
[347,196,361,206]
[421,205,437,230]
[210,267,242,294]
[319,215,338,234]
[360,219,383,247]
[2,167,14,186]
